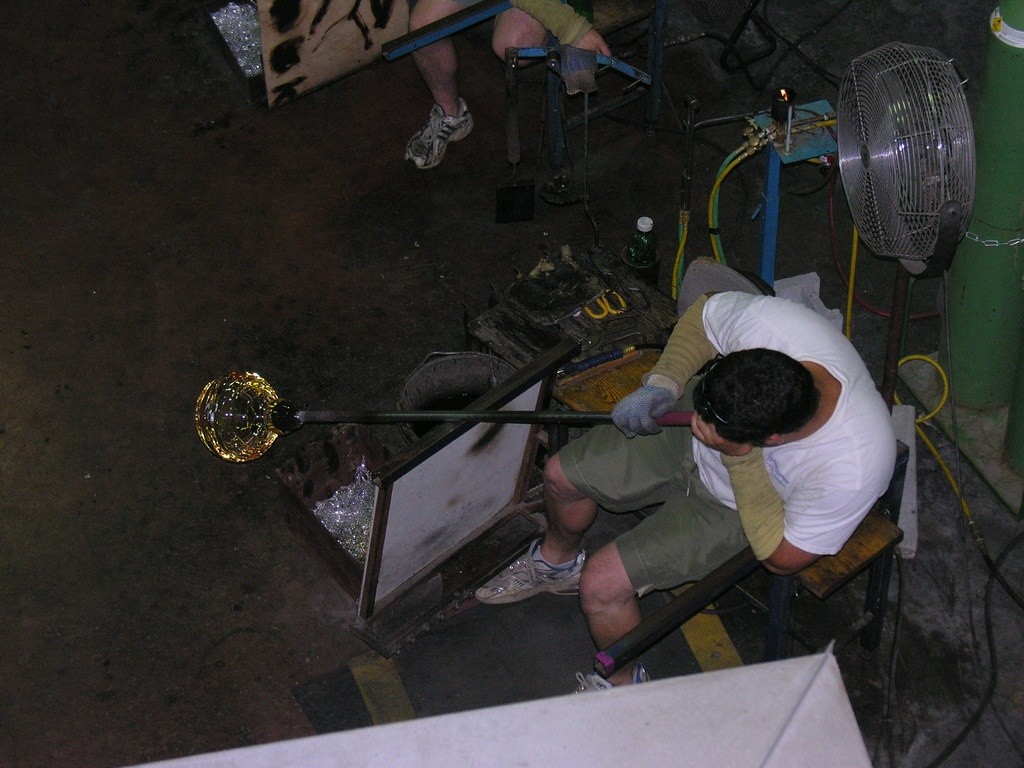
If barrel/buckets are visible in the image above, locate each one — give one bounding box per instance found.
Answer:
[397,351,518,447]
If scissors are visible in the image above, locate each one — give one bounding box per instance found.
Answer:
[581,315,648,351]
[590,254,651,312]
[570,259,627,319]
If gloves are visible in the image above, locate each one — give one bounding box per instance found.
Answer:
[611,374,679,438]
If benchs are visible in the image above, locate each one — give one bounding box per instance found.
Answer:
[552,353,904,662]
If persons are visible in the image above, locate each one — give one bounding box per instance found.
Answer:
[403,0,611,174]
[470,292,898,697]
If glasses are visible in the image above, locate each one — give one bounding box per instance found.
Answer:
[700,352,773,436]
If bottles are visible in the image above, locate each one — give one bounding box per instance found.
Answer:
[628,217,654,265]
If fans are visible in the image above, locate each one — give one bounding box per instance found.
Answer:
[836,42,977,559]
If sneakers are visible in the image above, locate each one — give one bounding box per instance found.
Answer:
[571,661,650,693]
[405,97,473,168]
[475,538,586,604]
